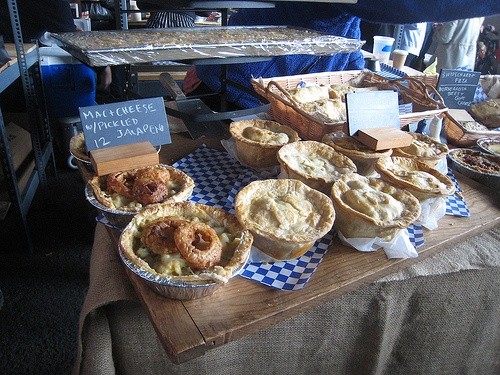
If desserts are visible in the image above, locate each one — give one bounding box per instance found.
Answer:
[70,112,455,301]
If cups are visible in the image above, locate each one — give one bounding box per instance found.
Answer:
[372,35,396,64]
[393,49,409,69]
[82,10,89,19]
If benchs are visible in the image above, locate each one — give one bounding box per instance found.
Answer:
[138,72,187,80]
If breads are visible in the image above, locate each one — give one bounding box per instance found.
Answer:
[289,83,360,121]
[471,98,500,127]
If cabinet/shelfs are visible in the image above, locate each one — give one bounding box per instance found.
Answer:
[117,0,404,103]
[0,0,59,252]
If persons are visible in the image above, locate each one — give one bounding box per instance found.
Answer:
[0,0,112,115]
[147,9,500,114]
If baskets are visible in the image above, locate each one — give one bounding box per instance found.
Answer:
[408,75,500,148]
[250,69,450,144]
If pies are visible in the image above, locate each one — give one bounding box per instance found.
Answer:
[448,137,500,184]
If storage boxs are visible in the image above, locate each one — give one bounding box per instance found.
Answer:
[38,45,97,119]
[5,122,36,193]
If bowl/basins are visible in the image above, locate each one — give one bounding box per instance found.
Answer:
[68,119,500,300]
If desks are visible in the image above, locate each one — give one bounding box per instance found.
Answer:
[128,17,221,26]
